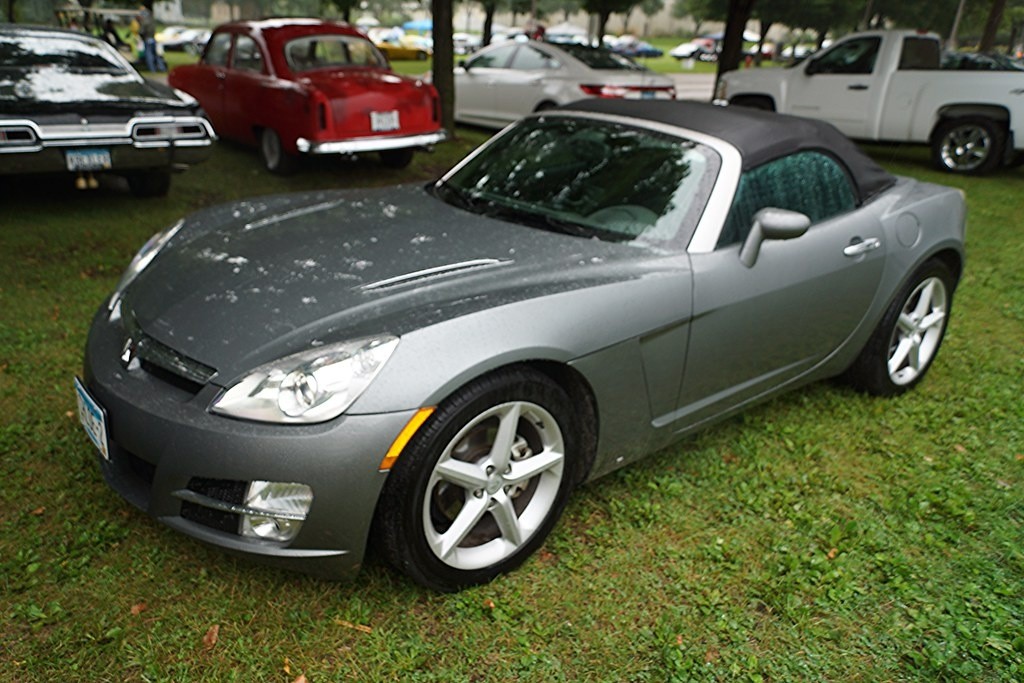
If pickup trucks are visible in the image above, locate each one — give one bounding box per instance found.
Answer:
[715,30,1024,177]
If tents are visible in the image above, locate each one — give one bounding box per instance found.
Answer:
[706,28,761,54]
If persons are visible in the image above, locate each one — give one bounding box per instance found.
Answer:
[525,20,546,42]
[67,3,167,72]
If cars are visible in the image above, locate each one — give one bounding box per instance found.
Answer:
[0,22,219,199]
[424,40,676,130]
[158,11,822,72]
[168,17,446,175]
[74,98,966,595]
